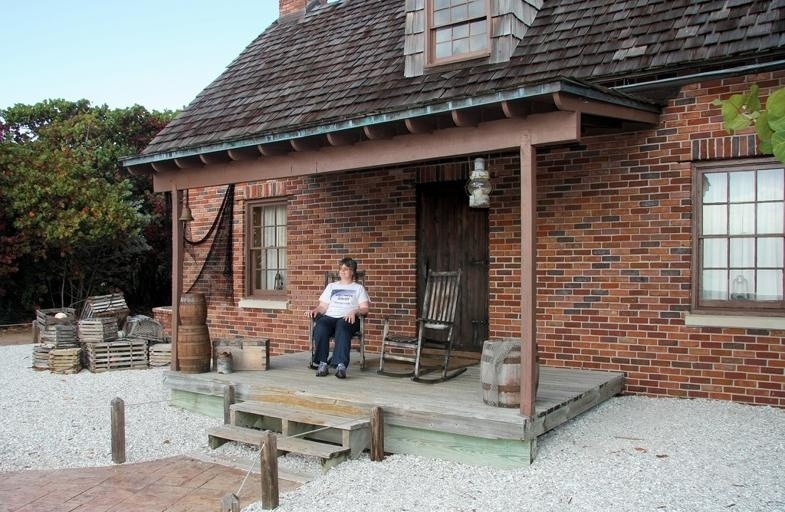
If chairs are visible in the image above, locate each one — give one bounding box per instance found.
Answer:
[376,269,467,384]
[308,270,366,372]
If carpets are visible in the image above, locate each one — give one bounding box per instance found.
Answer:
[355,355,480,371]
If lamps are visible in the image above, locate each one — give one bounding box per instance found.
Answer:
[465,152,492,208]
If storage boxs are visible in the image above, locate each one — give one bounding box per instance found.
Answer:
[212,337,269,371]
[33,292,172,374]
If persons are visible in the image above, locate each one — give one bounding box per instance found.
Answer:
[304,258,372,378]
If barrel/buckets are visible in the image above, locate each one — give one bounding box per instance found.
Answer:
[481,339,540,408]
[179,293,207,325]
[176,324,211,374]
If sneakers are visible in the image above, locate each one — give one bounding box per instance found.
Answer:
[316,362,330,377]
[334,364,347,378]
